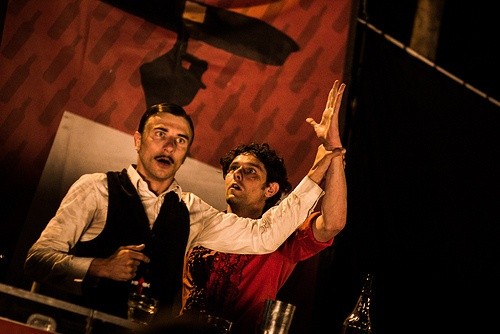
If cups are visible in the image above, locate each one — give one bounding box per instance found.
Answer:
[262,300,296,334]
[128,293,160,326]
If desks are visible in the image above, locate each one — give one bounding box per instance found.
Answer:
[0,282,133,334]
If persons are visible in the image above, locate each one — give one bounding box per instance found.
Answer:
[177,79,347,334]
[25,103,346,334]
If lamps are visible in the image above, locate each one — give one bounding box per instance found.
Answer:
[138,32,209,107]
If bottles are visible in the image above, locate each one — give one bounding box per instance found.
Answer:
[338,272,377,334]
